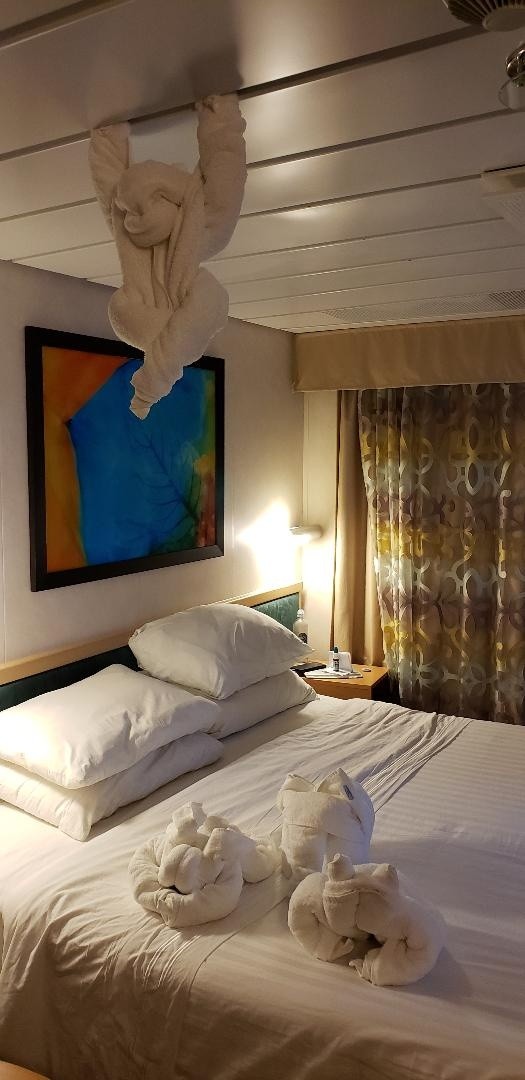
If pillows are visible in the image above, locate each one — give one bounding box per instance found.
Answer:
[1,663,223,842]
[130,602,317,742]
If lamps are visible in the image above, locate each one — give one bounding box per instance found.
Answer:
[291,524,323,540]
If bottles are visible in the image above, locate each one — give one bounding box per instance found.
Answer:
[333,646,340,672]
[292,608,309,665]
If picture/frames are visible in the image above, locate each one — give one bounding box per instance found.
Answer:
[24,324,225,593]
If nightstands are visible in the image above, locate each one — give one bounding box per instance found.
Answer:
[298,660,390,700]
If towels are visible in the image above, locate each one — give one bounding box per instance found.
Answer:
[87,91,252,420]
[276,767,448,987]
[129,799,278,929]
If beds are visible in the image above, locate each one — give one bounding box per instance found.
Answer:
[1,581,525,1080]
